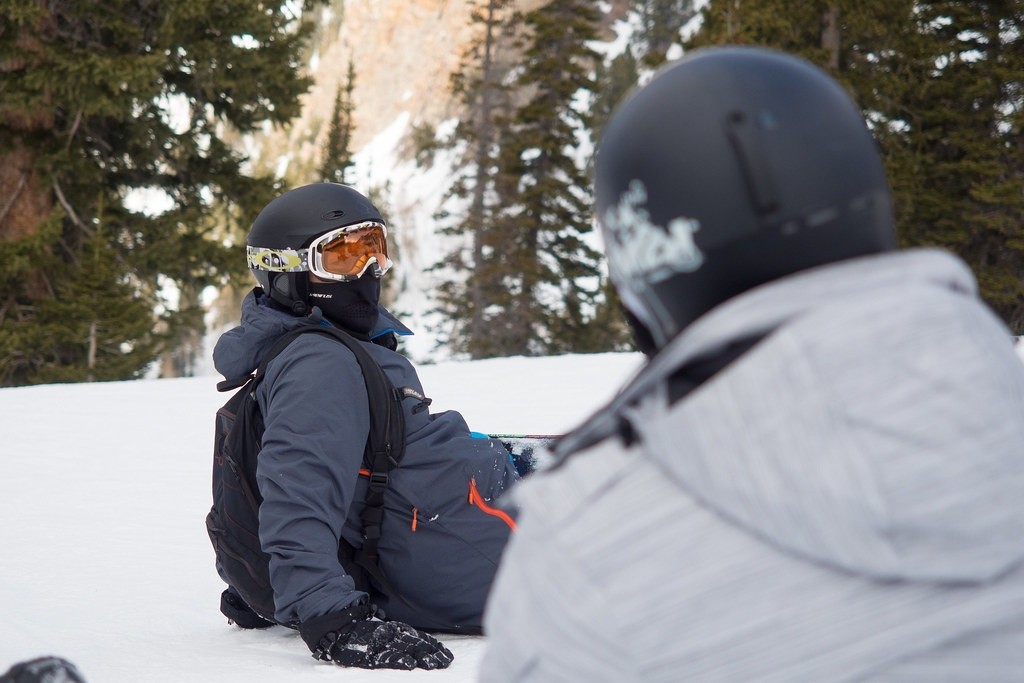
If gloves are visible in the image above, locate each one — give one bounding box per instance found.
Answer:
[314,611,455,672]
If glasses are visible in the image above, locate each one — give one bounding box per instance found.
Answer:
[247,222,394,281]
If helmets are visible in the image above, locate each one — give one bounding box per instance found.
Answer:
[244,183,393,310]
[592,45,898,355]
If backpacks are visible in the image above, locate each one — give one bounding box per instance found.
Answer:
[203,318,435,627]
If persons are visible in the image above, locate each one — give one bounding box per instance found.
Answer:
[0,44,1024,683]
[213,175,550,670]
